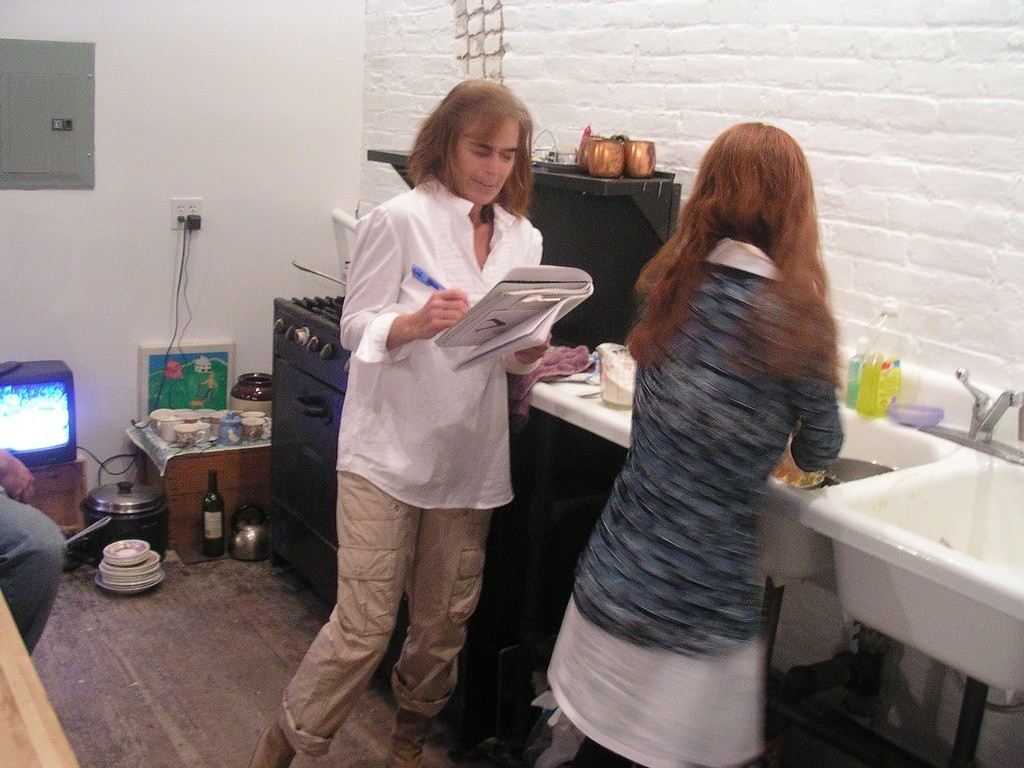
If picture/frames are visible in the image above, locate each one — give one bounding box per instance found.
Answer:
[136,341,236,423]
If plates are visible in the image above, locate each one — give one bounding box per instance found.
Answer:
[95,550,166,595]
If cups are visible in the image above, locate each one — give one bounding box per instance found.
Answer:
[576,134,657,179]
[595,341,636,411]
[148,407,266,448]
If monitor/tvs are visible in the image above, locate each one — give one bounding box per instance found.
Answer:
[0,360,77,467]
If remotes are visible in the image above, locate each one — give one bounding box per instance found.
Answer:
[0,360,21,375]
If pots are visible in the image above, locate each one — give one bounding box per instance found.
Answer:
[79,481,168,560]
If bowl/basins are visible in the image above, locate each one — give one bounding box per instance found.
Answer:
[103,539,150,566]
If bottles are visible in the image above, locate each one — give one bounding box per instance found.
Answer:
[202,470,225,558]
[846,295,903,418]
[228,373,274,418]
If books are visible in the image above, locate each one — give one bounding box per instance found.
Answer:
[434,266,594,375]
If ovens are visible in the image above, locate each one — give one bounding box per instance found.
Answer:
[273,355,350,616]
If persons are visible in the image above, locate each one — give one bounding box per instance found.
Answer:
[0,450,66,655]
[247,82,551,767]
[532,123,845,768]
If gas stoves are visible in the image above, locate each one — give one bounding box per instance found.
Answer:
[271,296,353,394]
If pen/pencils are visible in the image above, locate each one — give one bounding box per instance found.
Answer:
[411,265,469,313]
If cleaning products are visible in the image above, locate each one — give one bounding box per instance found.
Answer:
[854,300,901,420]
[845,337,870,408]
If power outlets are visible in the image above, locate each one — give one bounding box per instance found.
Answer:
[170,198,200,230]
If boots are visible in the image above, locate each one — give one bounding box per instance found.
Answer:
[249,713,297,768]
[387,706,434,768]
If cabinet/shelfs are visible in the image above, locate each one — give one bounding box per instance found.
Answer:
[365,148,683,354]
[21,452,87,537]
[140,427,272,550]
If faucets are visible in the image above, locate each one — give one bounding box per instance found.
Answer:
[956,366,1022,443]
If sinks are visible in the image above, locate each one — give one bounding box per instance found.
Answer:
[770,410,940,503]
[816,456,1024,586]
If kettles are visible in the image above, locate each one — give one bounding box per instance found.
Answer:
[229,502,272,561]
